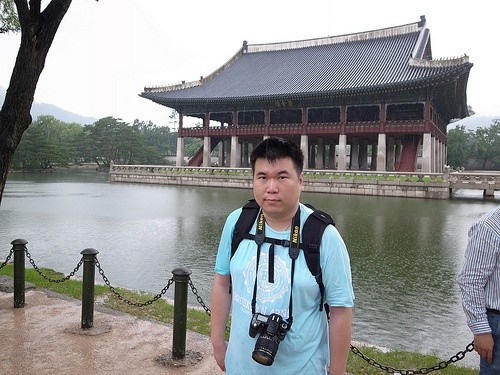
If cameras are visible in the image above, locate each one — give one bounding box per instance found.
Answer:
[249,312,293,366]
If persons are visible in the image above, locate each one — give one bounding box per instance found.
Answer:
[454,204,500,375]
[208,136,357,374]
